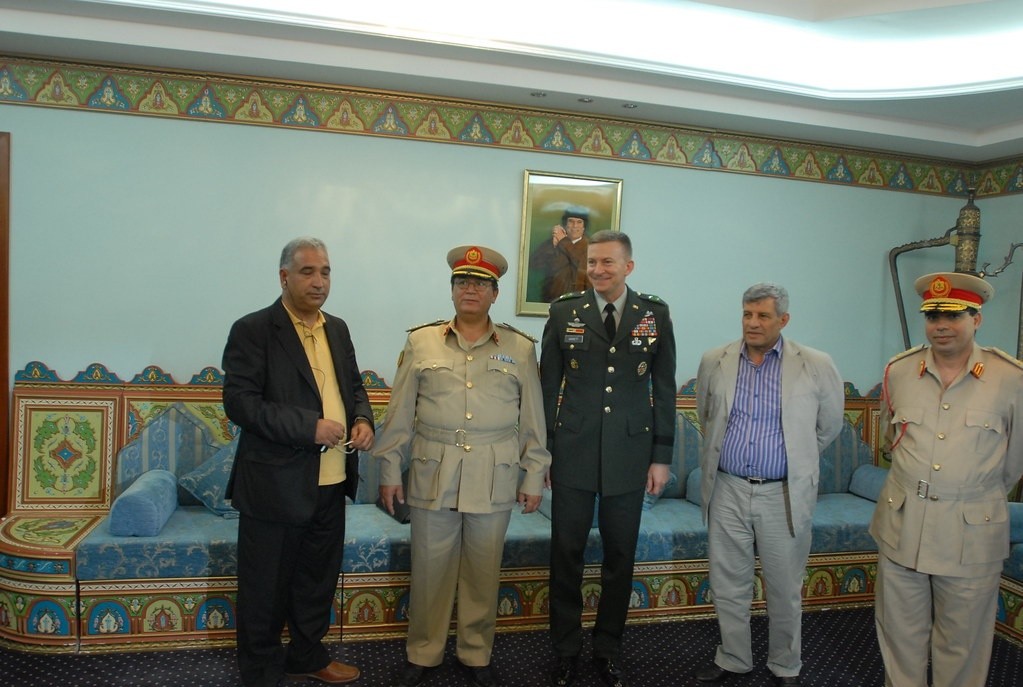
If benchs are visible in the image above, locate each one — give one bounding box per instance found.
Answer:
[1,362,1023,647]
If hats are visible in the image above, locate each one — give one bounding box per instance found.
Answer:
[446,244,509,285]
[913,271,994,314]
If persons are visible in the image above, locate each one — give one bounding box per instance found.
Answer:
[221,237,375,687]
[376,244,551,687]
[867,271,1023,687]
[541,231,678,687]
[691,284,846,687]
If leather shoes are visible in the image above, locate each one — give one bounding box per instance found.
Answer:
[594,654,625,687]
[774,672,801,686]
[694,658,731,684]
[285,647,361,683]
[398,657,425,687]
[549,654,577,687]
[456,656,495,687]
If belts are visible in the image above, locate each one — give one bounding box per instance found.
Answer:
[736,474,789,486]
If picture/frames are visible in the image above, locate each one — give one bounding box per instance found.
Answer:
[514,169,623,316]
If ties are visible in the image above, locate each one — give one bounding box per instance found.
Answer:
[602,302,616,341]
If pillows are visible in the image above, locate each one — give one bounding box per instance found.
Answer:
[175,427,243,519]
[108,468,178,537]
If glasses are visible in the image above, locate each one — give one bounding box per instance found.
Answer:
[453,280,494,291]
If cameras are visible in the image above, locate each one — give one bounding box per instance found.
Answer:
[321,445,328,453]
